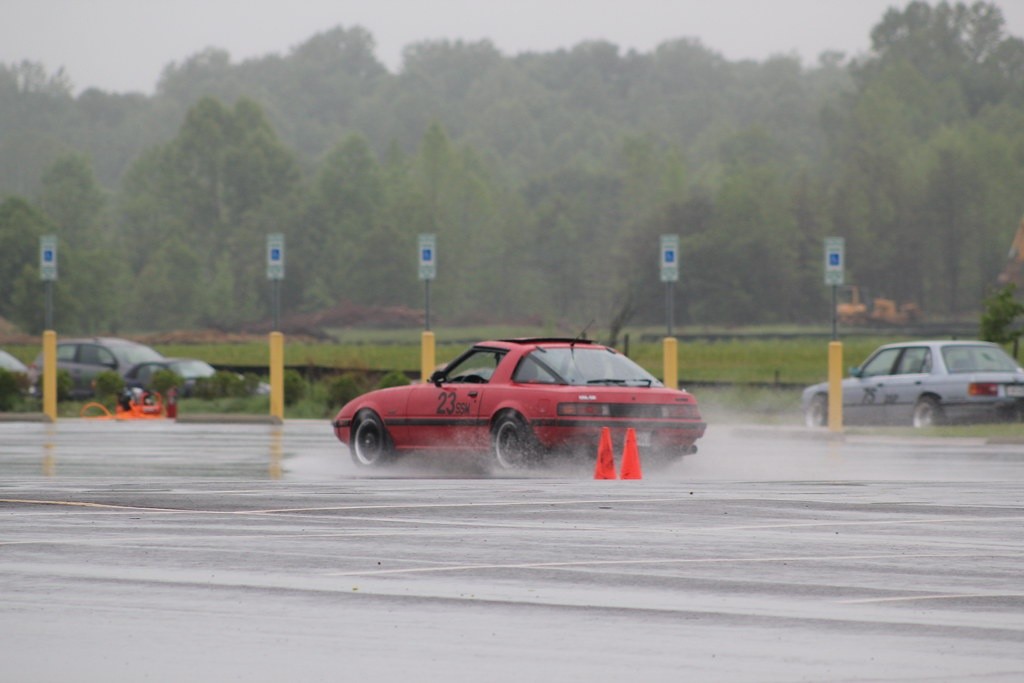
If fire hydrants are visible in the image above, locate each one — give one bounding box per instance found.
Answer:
[166,386,181,418]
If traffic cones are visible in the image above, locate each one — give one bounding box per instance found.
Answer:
[593,426,618,481]
[615,426,645,481]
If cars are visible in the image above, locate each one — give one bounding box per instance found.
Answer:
[798,339,1024,427]
[29,338,166,403]
[0,350,43,412]
[329,335,708,477]
[117,355,273,412]
